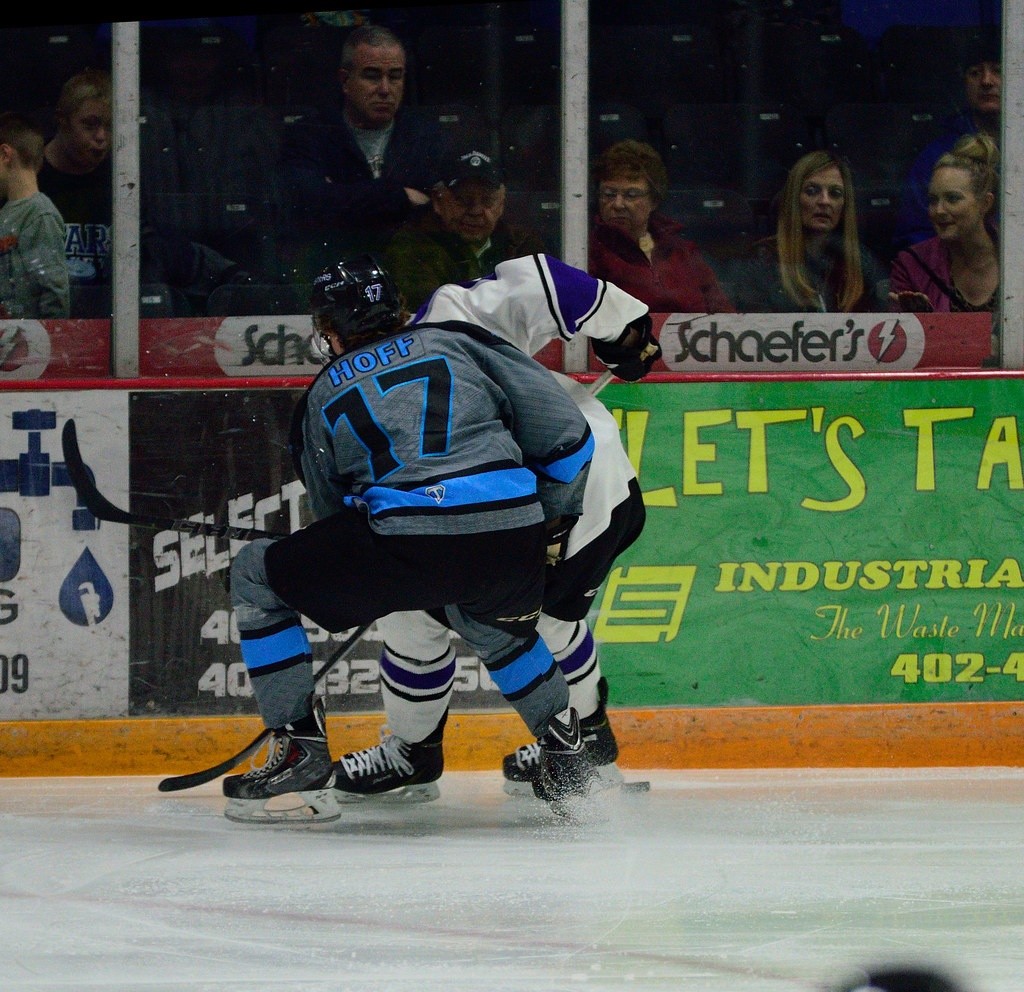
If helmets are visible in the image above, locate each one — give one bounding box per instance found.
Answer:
[310,248,402,358]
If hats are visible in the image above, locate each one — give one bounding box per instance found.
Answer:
[445,144,495,179]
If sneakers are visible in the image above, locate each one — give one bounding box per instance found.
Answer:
[503,676,650,799]
[222,692,342,826]
[331,706,450,805]
[532,706,603,826]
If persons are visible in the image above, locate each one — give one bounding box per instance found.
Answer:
[0,20,1005,319]
[223,214,664,832]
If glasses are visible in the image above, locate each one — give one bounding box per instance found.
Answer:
[597,187,652,201]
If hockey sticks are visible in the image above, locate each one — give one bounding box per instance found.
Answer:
[61,418,292,542]
[157,619,374,794]
